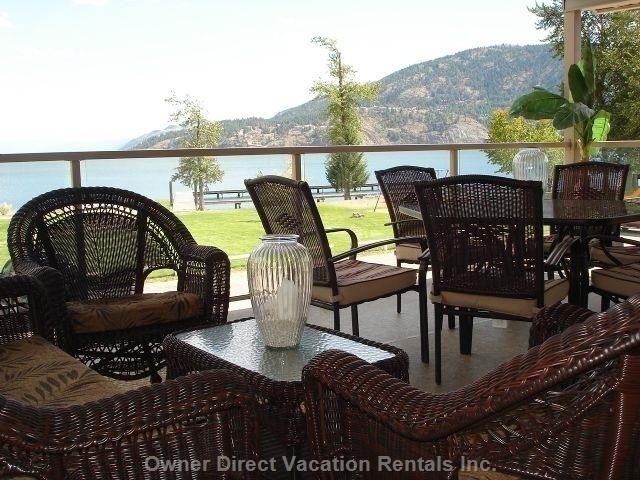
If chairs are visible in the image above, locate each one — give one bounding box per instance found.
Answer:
[3,158,636,479]
